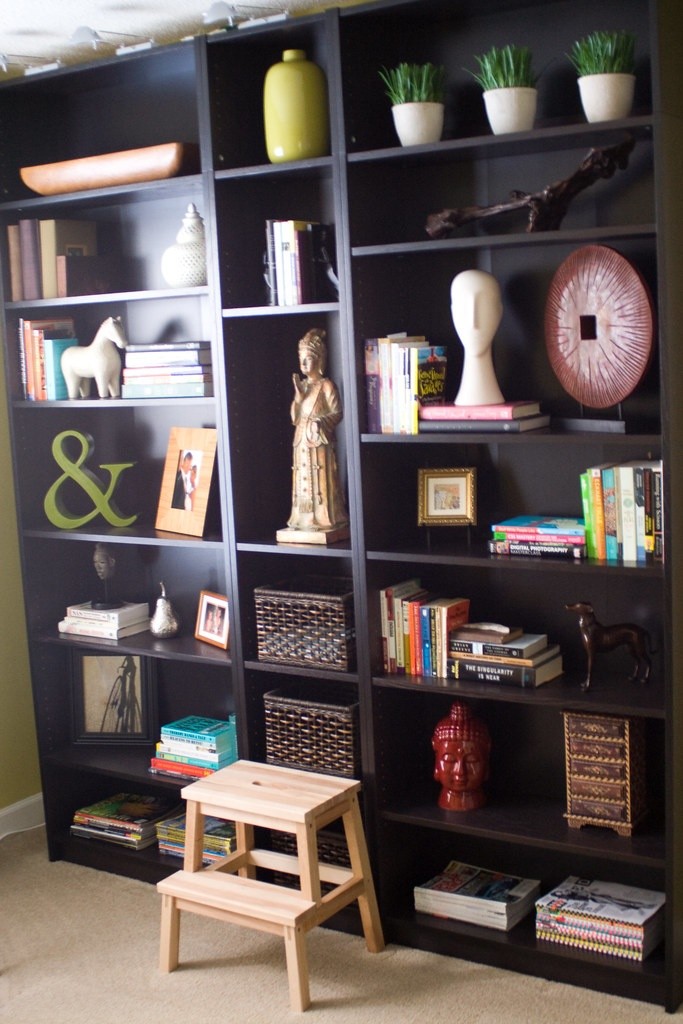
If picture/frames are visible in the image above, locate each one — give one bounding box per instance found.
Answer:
[195,590,232,650]
[418,468,478,527]
[67,649,160,746]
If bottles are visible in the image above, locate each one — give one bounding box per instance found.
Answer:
[264,50,331,163]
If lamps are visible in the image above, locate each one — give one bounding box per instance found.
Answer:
[69,26,160,57]
[202,1,289,31]
[0,53,63,77]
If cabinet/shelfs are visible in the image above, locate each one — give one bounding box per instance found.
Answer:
[0,1,682,1014]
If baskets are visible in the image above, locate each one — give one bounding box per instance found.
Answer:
[262,688,358,782]
[253,575,352,670]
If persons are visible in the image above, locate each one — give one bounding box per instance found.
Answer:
[172,453,198,511]
[431,701,490,812]
[92,544,116,580]
[205,609,223,635]
[287,330,348,532]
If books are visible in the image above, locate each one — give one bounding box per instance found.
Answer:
[363,332,551,436]
[488,458,665,563]
[379,576,567,690]
[66,714,235,867]
[58,598,151,640]
[8,219,214,401]
[262,220,335,306]
[412,861,668,960]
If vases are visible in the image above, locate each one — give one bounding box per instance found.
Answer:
[154,427,217,537]
[264,50,330,164]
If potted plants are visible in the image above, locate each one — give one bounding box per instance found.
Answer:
[566,28,638,124]
[461,43,556,136]
[378,64,445,148]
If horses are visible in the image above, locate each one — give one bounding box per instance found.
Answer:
[60,316,127,400]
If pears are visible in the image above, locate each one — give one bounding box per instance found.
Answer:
[148,582,183,638]
